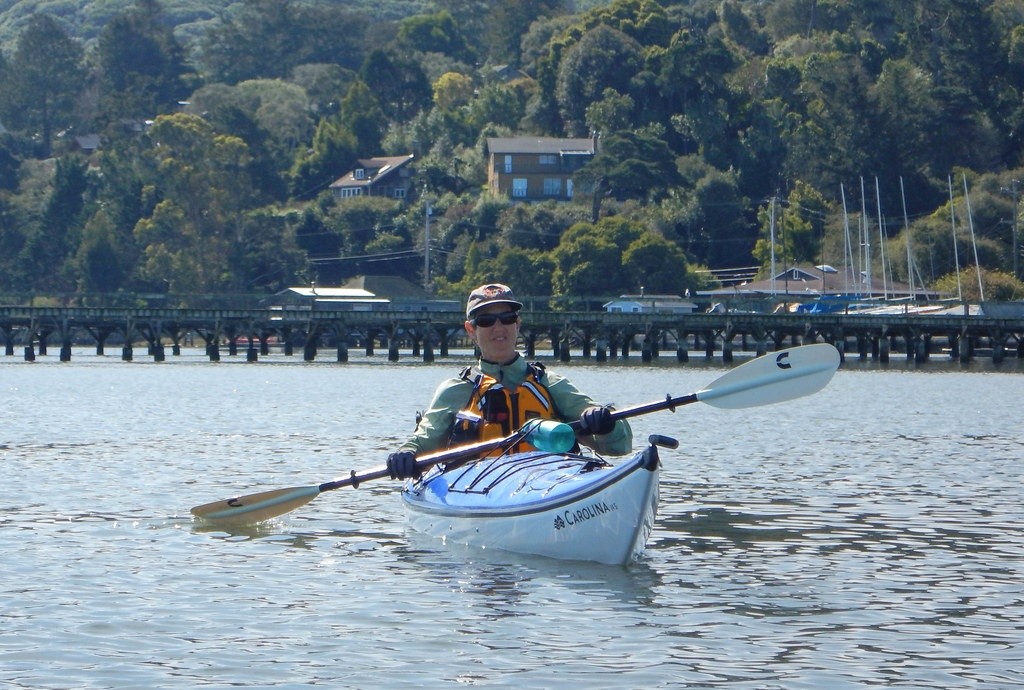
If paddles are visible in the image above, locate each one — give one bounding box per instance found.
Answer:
[190,342,841,525]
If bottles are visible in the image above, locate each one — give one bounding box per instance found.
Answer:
[522,418,575,454]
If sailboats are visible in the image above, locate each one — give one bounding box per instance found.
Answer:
[771,170,1024,349]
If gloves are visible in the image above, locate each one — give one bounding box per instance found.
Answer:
[575,405,616,437]
[387,453,422,482]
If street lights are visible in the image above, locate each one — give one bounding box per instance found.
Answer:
[421,184,430,304]
[771,197,784,297]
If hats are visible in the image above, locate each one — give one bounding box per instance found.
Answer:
[466,284,523,319]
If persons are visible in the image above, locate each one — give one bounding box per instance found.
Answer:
[387,284,633,481]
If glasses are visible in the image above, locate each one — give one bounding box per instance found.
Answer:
[468,311,518,328]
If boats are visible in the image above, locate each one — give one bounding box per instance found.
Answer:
[399,432,682,569]
